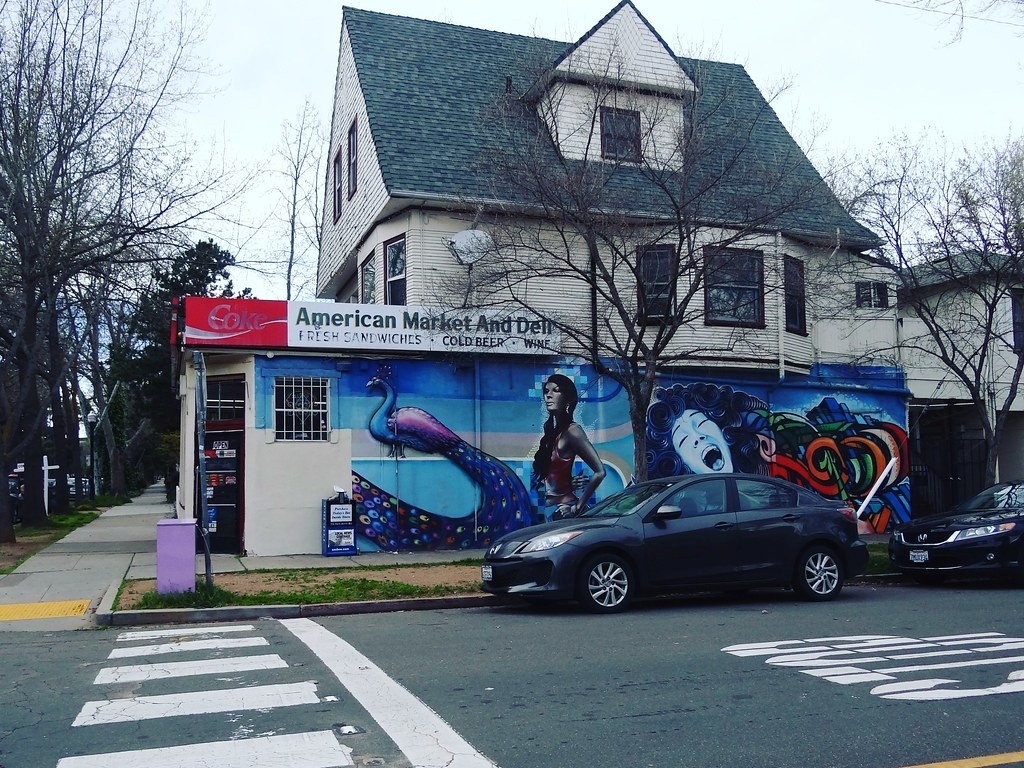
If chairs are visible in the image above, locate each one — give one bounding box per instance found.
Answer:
[680,495,698,517]
[763,495,780,510]
[699,484,724,515]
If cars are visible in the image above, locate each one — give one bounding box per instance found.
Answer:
[6,472,105,526]
[479,472,871,615]
[888,478,1024,588]
